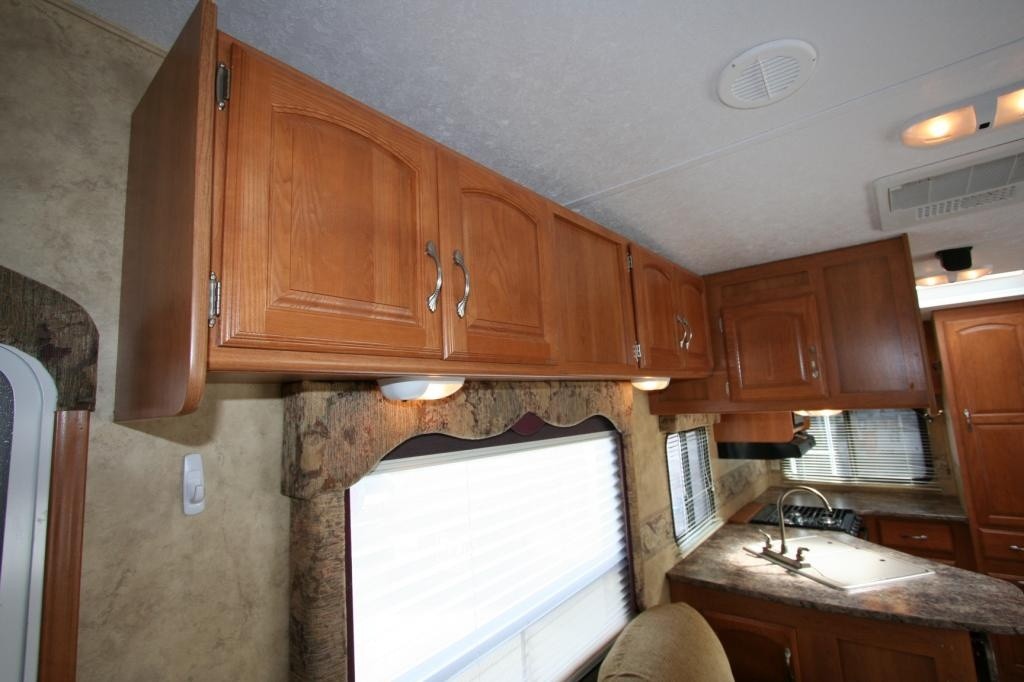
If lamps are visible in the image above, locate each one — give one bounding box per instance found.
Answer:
[898,79,1024,148]
[629,377,671,392]
[911,244,992,287]
[378,377,466,402]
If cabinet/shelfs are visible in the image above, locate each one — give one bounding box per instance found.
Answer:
[113,0,714,424]
[931,301,1024,682]
[648,233,938,420]
[861,517,975,574]
[668,581,977,682]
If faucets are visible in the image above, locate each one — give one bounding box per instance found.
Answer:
[758,486,832,568]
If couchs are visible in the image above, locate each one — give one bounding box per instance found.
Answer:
[596,602,736,682]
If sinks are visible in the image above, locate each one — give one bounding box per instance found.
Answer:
[743,535,936,591]
